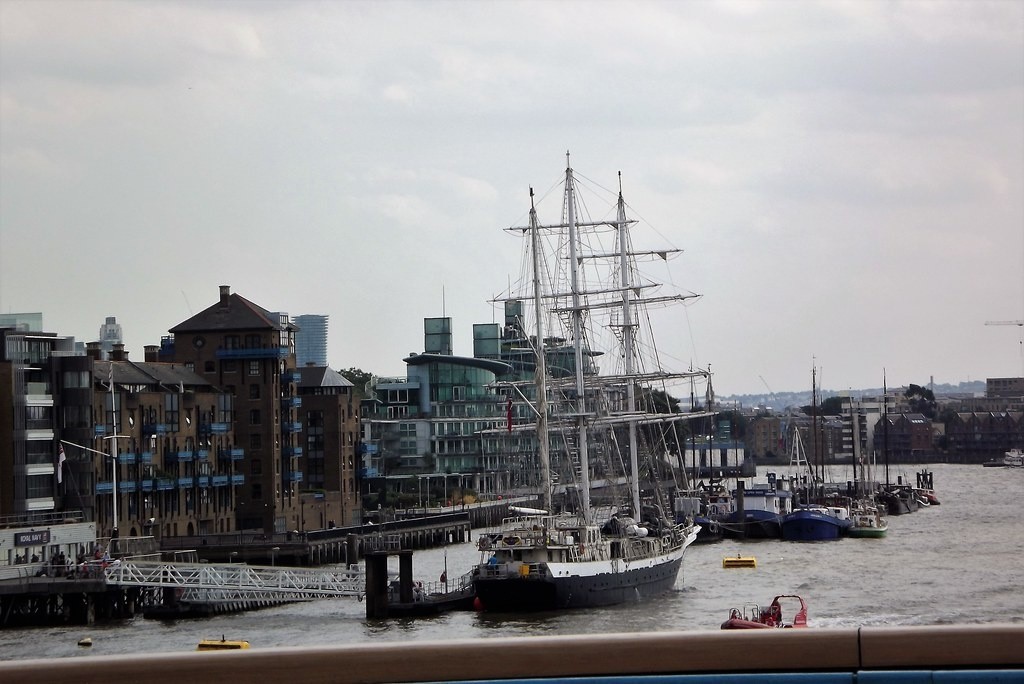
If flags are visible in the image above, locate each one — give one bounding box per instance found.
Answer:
[58,442,66,483]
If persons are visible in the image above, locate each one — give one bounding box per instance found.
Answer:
[487,554,497,576]
[14,545,111,580]
[610,542,620,558]
[731,609,738,619]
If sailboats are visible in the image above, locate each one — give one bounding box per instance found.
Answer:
[473,152,720,615]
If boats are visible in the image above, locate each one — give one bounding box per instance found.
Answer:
[721,595,808,630]
[983,449,1024,467]
[677,368,940,541]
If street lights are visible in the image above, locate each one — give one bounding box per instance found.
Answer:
[100,360,131,560]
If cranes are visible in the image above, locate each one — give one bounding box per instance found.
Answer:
[984,320,1024,346]
